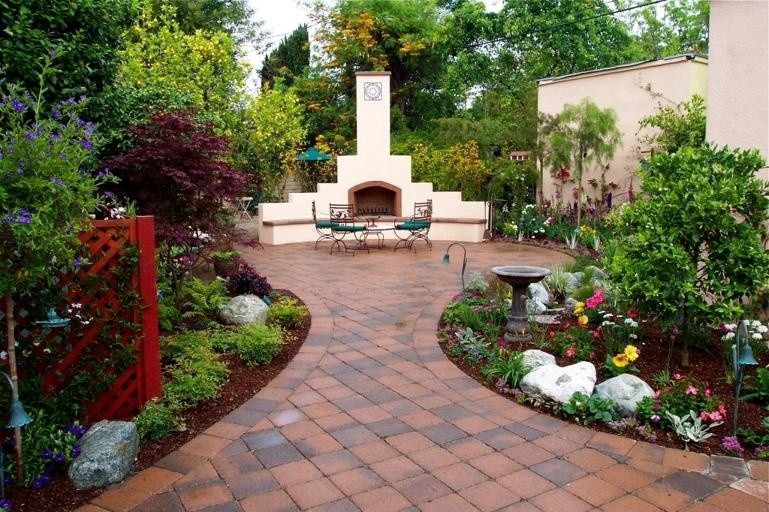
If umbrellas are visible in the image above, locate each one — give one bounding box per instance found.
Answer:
[292,147,329,161]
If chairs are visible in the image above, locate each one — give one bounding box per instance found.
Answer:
[310,199,433,257]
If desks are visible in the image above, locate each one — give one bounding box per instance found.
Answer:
[224,197,254,220]
